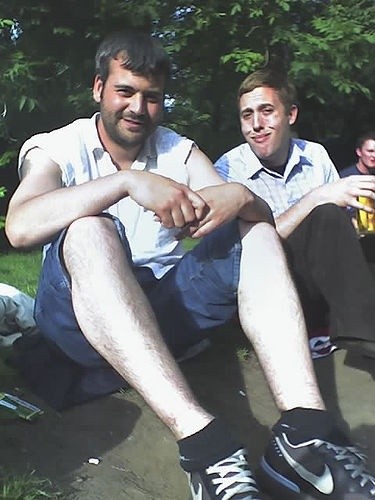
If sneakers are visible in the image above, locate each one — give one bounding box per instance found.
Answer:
[180,449,267,500]
[258,422,375,499]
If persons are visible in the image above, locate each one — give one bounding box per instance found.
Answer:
[3,27,374,500]
[339,133,374,265]
[213,68,375,381]
